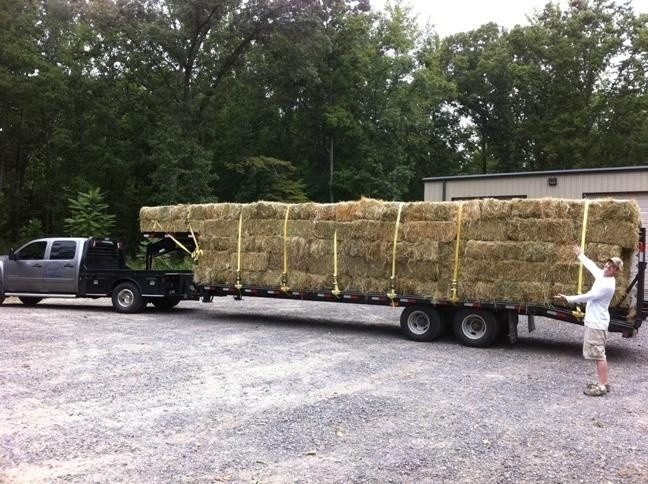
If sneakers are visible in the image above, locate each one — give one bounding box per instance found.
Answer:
[583,383,609,396]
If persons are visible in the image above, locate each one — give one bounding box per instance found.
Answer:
[553,244,624,396]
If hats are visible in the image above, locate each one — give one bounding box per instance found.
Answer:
[607,257,623,272]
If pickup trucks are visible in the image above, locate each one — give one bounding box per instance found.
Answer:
[0,235,213,313]
